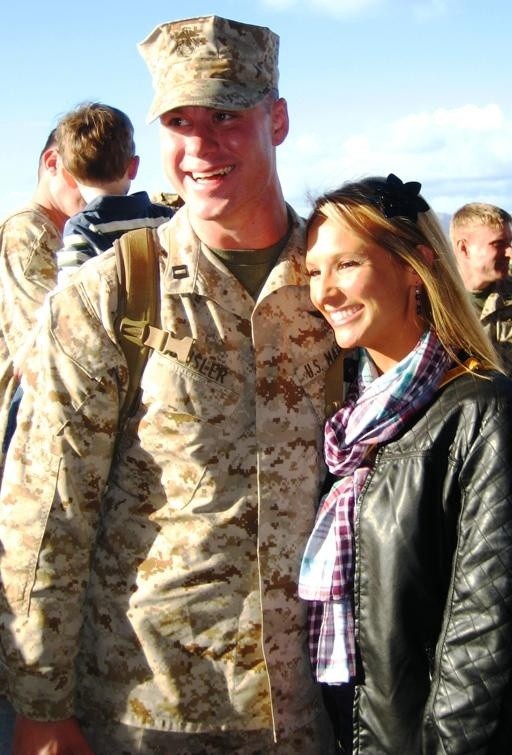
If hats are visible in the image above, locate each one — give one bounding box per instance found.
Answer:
[136,13,281,125]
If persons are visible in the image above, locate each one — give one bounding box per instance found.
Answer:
[449,202,512,374]
[0,128,86,492]
[299,174,512,755]
[54,99,174,287]
[0,15,359,755]
